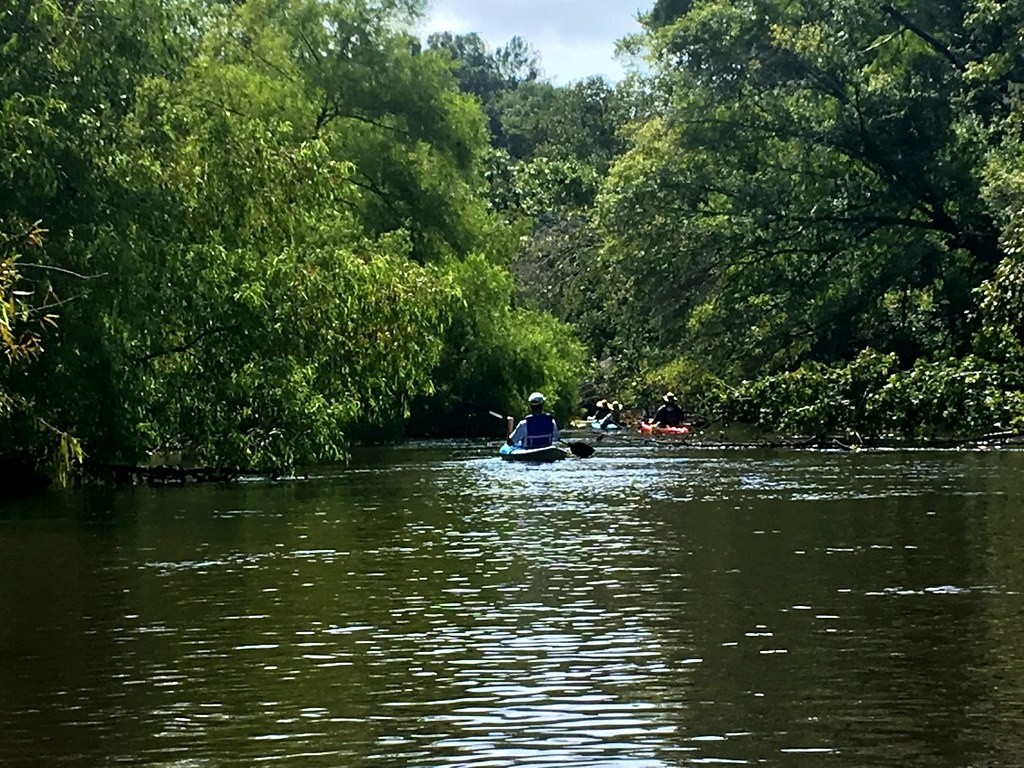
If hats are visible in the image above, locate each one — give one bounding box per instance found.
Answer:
[596,399,612,410]
[607,400,623,411]
[528,391,546,406]
[663,392,677,402]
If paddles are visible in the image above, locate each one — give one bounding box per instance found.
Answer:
[486,409,596,459]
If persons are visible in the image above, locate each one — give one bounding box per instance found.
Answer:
[506,392,561,450]
[652,392,685,429]
[587,399,623,424]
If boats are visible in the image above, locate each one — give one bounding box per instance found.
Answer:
[499,440,567,463]
[570,419,586,428]
[591,423,631,432]
[637,420,688,437]
